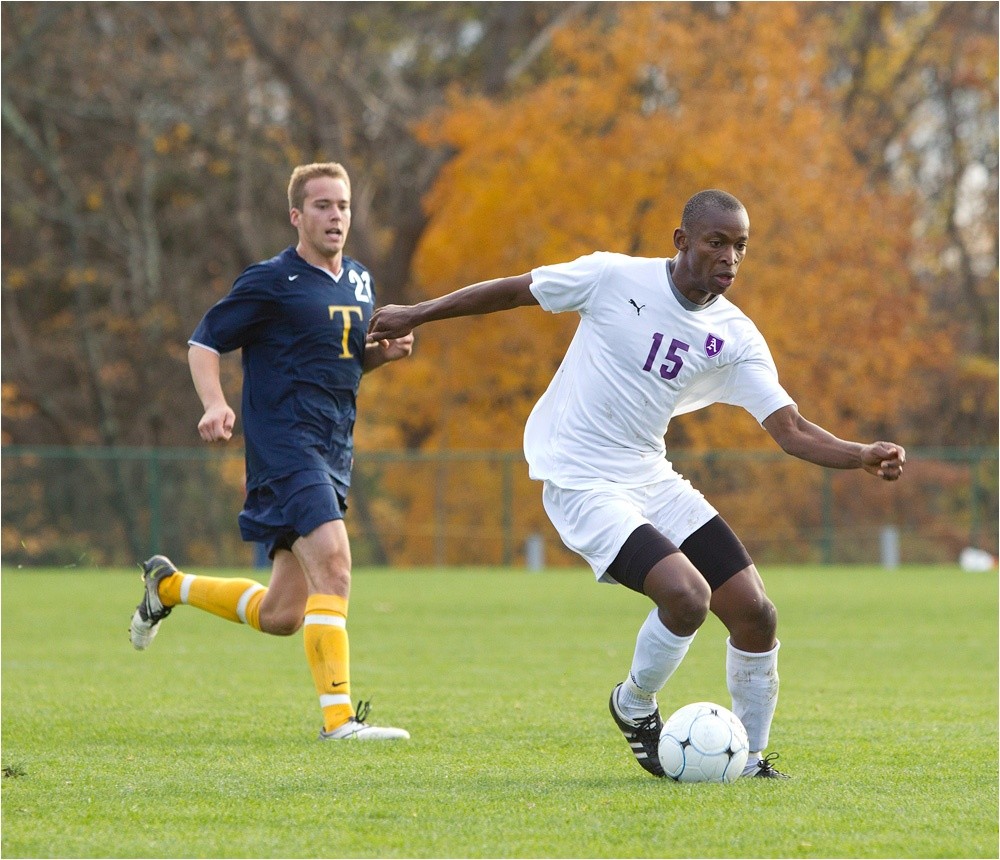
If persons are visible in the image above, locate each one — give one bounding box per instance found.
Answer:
[128,162,414,744]
[367,189,905,780]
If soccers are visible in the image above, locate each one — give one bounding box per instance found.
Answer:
[656,701,750,783]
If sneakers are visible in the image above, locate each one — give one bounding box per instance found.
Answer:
[740,752,791,782]
[609,682,665,778]
[317,701,411,742]
[128,555,178,650]
